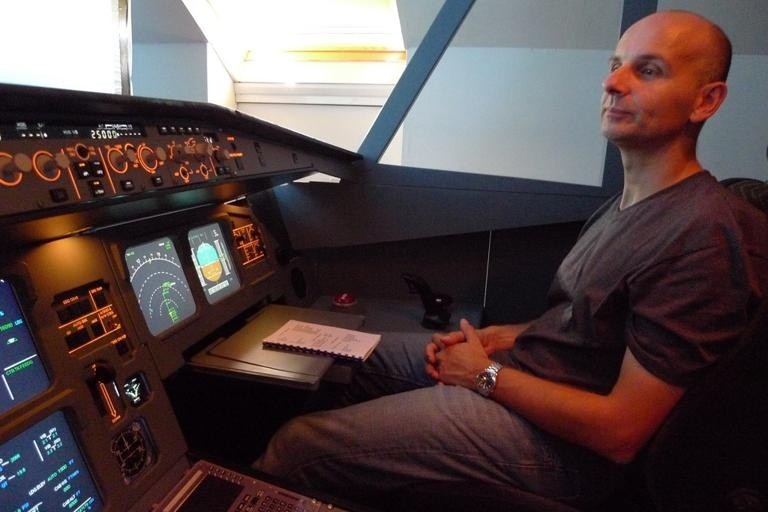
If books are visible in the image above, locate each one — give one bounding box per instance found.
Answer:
[261,318,382,362]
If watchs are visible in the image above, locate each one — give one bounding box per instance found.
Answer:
[475,360,504,396]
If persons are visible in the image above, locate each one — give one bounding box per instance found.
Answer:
[241,8,768,510]
